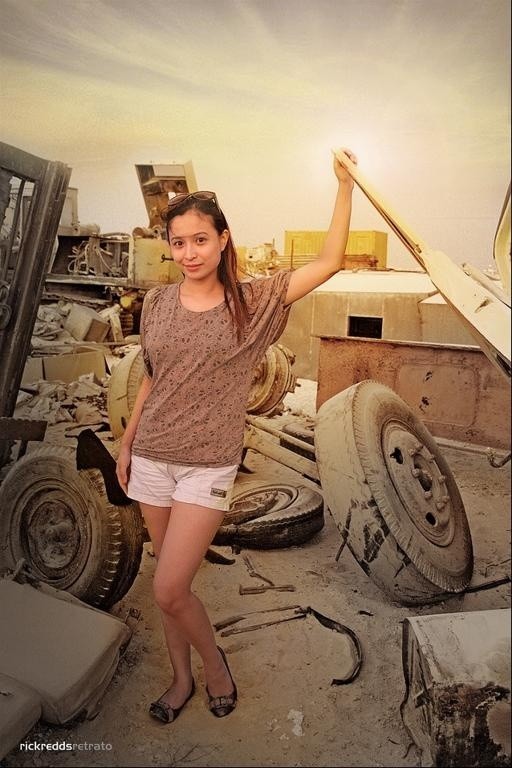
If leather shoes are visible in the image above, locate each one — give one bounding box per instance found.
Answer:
[149,673,194,723]
[206,644,237,718]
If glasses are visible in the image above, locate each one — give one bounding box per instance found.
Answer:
[167,190,221,213]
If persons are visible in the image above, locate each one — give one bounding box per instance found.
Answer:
[112,145,362,726]
